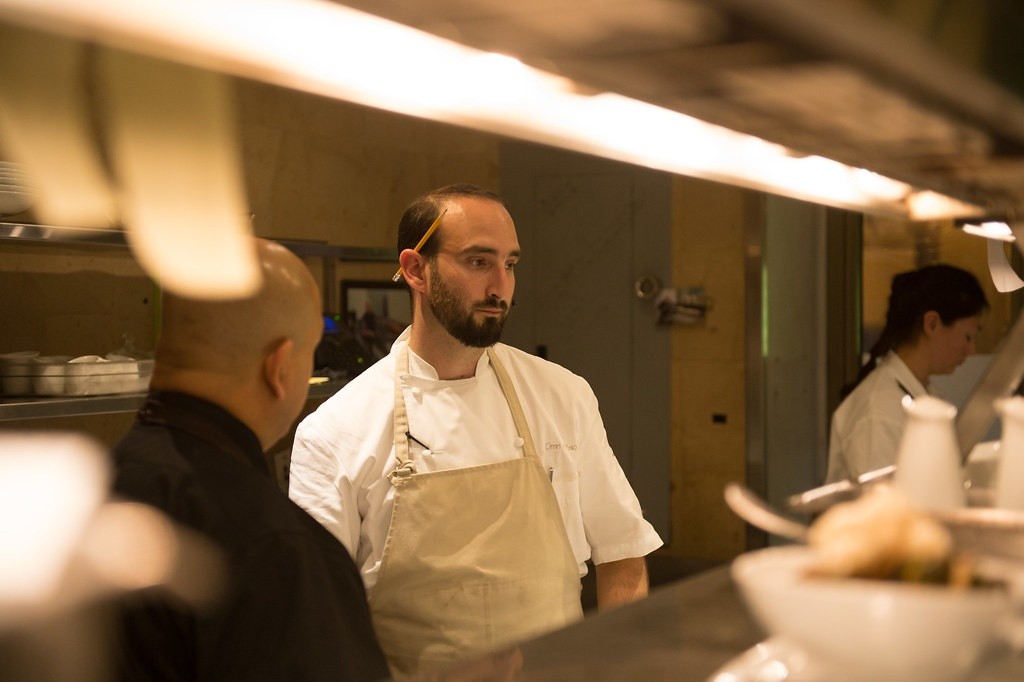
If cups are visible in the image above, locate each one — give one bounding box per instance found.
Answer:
[33,355,73,395]
[106,354,137,380]
[68,355,106,396]
[0,351,39,394]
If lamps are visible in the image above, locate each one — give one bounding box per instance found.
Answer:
[631,274,706,328]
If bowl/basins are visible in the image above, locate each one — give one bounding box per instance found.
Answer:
[0,161,37,216]
[728,545,1022,682]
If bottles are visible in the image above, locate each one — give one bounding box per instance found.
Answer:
[895,396,964,510]
[992,397,1024,515]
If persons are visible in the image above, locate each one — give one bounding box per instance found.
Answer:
[102,239,392,682]
[825,262,990,482]
[288,185,664,682]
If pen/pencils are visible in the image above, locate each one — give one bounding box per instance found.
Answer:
[392,208,450,283]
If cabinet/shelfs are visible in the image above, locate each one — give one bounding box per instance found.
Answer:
[0,217,160,423]
